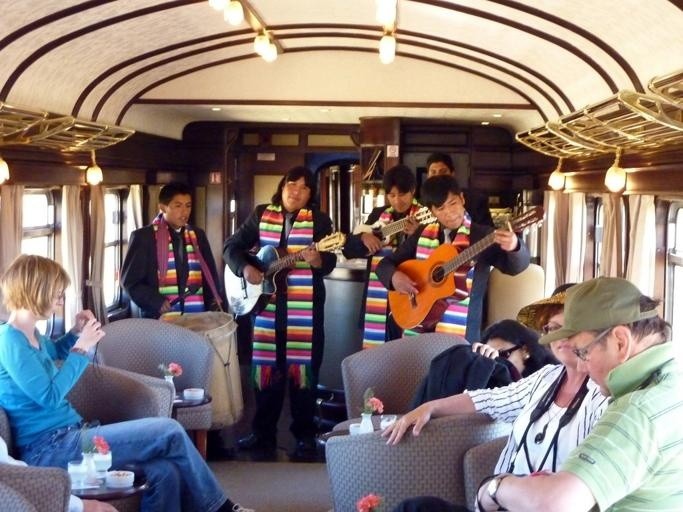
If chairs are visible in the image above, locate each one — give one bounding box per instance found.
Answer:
[314,267,367,424]
[90,318,212,467]
[328,333,514,512]
[463,437,511,510]
[0,362,172,512]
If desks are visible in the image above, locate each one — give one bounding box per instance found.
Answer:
[68,463,150,503]
[173,393,211,461]
[316,430,350,451]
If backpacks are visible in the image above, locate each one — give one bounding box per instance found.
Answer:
[410,344,511,411]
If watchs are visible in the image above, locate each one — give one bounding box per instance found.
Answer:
[488,475,507,504]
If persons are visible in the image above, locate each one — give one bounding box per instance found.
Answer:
[0,254,256,511]
[470,320,560,379]
[380,282,612,478]
[116,179,223,320]
[221,167,336,453]
[342,154,529,350]
[0,434,120,511]
[473,276,683,512]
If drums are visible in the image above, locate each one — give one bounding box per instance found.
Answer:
[159,312,243,430]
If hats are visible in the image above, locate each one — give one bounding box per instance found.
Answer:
[516,291,564,330]
[538,277,660,346]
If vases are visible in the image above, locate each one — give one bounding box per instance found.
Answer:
[164,377,177,400]
[360,413,374,434]
[79,453,96,480]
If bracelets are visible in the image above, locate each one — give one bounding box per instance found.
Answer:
[69,347,89,358]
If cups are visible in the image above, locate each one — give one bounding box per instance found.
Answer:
[90,453,112,480]
[378,412,398,428]
[349,421,368,436]
[65,459,88,491]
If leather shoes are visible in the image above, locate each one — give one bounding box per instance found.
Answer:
[296,439,325,455]
[238,433,279,448]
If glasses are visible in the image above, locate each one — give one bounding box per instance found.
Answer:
[501,345,521,357]
[573,328,612,360]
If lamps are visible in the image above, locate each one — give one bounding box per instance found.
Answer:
[547,153,567,190]
[602,149,628,194]
[203,1,287,65]
[85,149,103,185]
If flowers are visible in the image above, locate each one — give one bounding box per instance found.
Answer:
[81,433,108,456]
[362,387,384,416]
[154,363,181,376]
[355,493,383,512]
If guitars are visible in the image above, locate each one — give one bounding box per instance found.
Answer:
[388,207,546,330]
[353,209,437,257]
[224,231,346,317]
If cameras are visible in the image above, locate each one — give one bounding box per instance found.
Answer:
[80,319,100,330]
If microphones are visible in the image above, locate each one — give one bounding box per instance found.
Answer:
[170,283,200,307]
